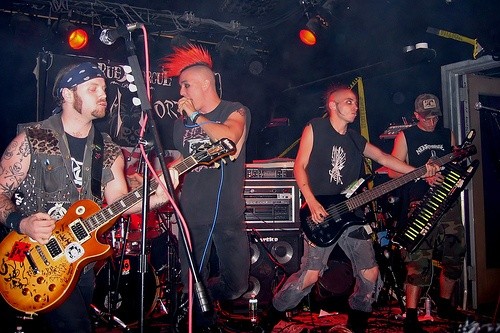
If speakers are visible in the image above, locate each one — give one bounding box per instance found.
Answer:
[211,222,304,313]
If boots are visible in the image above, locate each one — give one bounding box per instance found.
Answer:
[437,296,475,324]
[402,306,428,333]
[255,298,286,333]
[345,307,372,333]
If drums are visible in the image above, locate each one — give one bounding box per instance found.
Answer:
[95,253,161,326]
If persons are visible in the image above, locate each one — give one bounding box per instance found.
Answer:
[0,63,179,333]
[160,42,251,333]
[388,94,468,333]
[259,84,441,333]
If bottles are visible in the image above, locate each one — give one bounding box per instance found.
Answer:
[248,294,258,322]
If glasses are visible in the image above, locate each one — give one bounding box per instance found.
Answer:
[423,116,439,123]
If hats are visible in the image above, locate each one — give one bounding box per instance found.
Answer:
[415,93,442,118]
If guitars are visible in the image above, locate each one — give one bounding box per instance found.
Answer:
[300,142,478,248]
[0,136,236,315]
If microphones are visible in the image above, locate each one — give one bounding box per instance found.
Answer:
[100,23,144,45]
[182,110,188,126]
[474,102,500,113]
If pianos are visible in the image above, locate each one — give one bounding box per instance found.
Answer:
[395,130,480,255]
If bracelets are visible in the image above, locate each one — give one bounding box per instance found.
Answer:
[193,114,203,124]
[190,112,200,121]
[6,212,28,234]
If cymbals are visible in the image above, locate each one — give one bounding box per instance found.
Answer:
[156,210,175,215]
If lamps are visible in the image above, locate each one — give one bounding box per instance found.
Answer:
[299,7,336,46]
[52,20,88,50]
[247,54,268,76]
